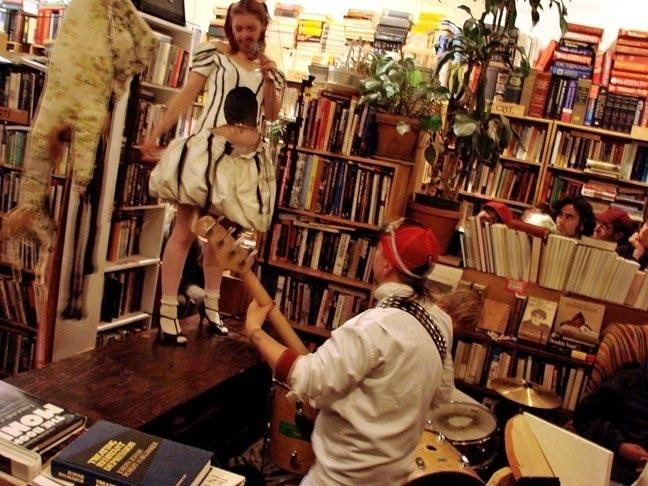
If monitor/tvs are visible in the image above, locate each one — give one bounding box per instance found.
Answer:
[523,412,614,486]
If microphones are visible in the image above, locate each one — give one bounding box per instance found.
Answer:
[247,40,282,85]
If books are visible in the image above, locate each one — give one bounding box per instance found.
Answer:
[1,0,647,486]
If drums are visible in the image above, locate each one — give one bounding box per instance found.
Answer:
[425,383,497,448]
[403,428,485,486]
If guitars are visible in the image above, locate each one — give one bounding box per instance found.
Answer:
[193,212,319,419]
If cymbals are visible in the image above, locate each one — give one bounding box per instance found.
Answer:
[490,373,563,411]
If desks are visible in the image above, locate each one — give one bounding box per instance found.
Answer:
[0,309,264,486]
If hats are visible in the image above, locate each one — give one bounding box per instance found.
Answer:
[595,207,631,227]
[381,217,441,280]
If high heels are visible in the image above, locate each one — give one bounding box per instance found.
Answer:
[158,315,188,347]
[198,302,229,335]
